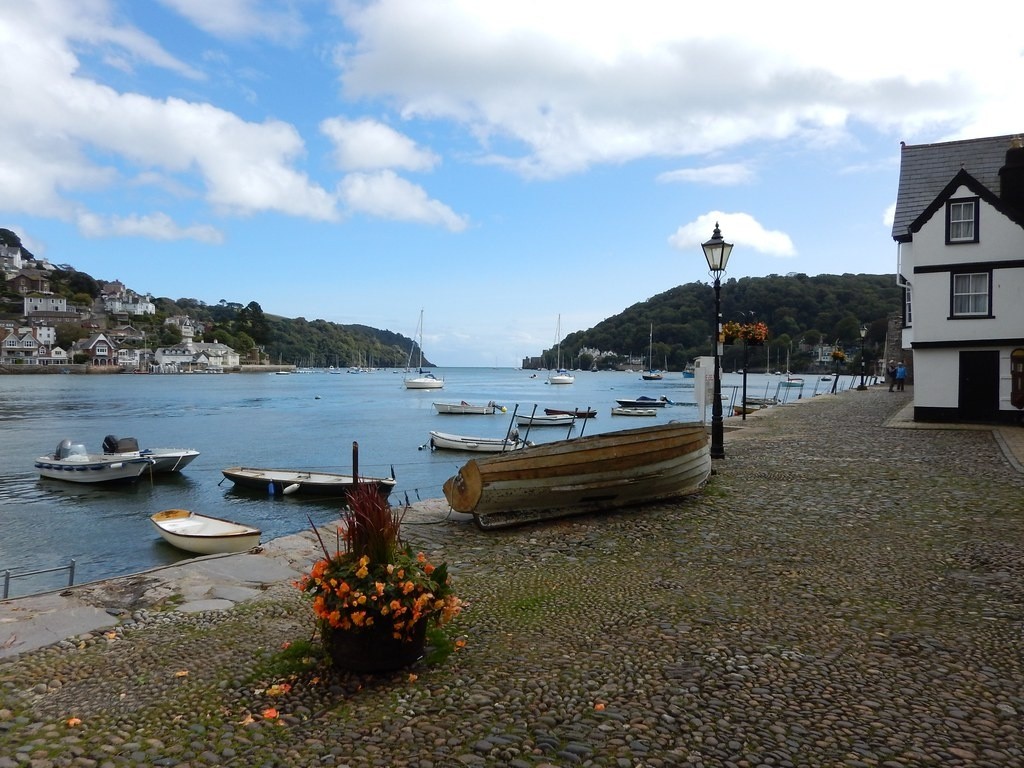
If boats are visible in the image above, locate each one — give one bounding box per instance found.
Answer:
[33,440,155,491]
[442,420,715,530]
[90,435,199,476]
[514,414,578,428]
[275,352,430,375]
[614,395,666,408]
[221,467,397,503]
[150,509,263,556]
[611,406,659,417]
[429,430,519,454]
[544,408,598,419]
[720,345,838,415]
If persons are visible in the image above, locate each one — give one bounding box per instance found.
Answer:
[886,360,907,393]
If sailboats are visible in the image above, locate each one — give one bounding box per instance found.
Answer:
[513,314,694,384]
[403,310,445,389]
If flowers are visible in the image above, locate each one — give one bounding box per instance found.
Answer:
[292,521,462,643]
[830,351,842,358]
[721,320,741,338]
[738,322,768,340]
[841,353,848,359]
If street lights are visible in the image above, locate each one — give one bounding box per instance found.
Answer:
[702,222,735,459]
[857,324,868,391]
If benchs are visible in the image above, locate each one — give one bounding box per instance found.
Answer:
[156,519,203,529]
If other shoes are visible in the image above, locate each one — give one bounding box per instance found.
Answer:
[888,390,894,392]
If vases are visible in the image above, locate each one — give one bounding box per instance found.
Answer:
[839,357,844,361]
[748,338,764,346]
[833,355,839,360]
[722,336,738,345]
[321,605,427,673]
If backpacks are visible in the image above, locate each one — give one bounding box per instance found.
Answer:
[887,366,894,376]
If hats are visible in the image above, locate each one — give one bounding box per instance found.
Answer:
[897,362,903,364]
[889,360,894,363]
